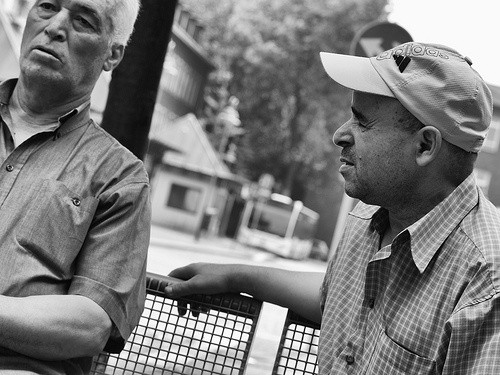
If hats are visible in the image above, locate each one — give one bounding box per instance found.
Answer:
[320,41,494,153]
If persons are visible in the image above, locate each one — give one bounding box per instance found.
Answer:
[165,41,500,375]
[0,0,152,375]
